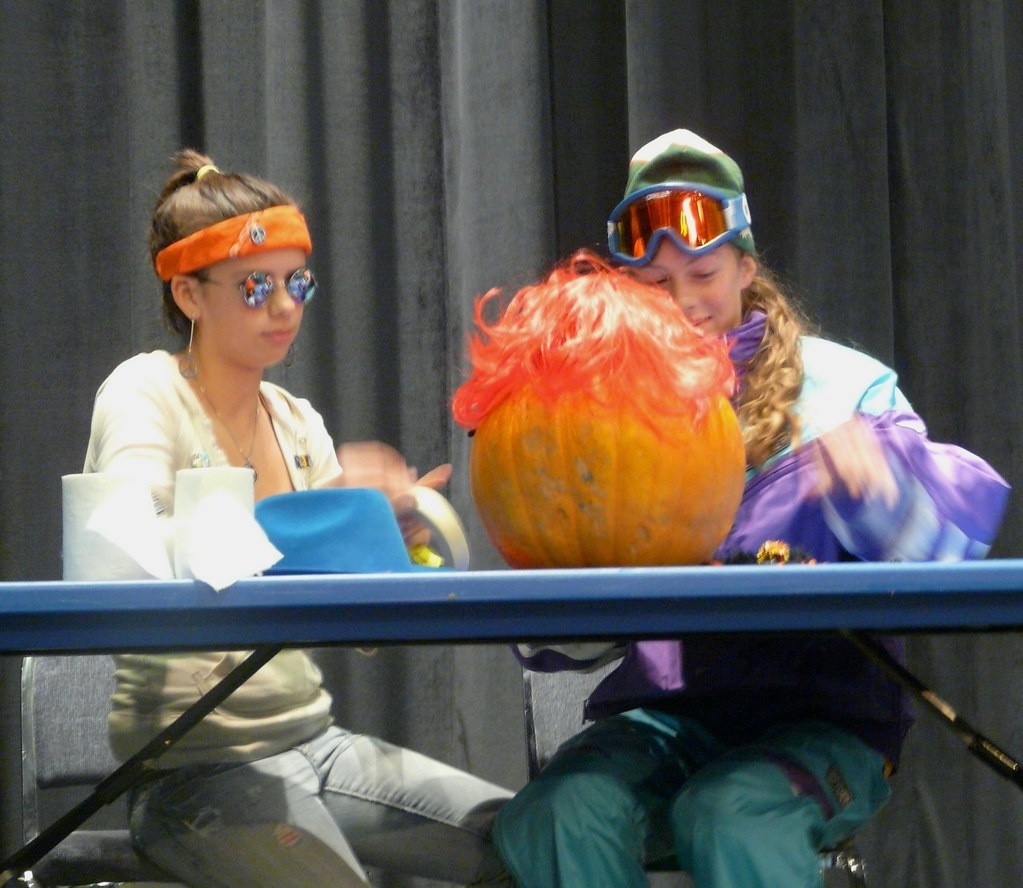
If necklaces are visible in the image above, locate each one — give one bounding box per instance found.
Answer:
[195,378,259,484]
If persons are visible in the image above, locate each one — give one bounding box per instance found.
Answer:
[80,145,525,888]
[484,125,1013,887]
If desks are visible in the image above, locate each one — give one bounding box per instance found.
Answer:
[0,557,1023,800]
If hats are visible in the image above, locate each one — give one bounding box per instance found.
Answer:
[624,129,755,257]
[254,487,455,575]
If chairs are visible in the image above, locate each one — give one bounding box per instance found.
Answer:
[512,644,869,888]
[21,653,176,888]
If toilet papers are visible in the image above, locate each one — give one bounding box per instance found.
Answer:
[171,465,284,593]
[60,465,174,584]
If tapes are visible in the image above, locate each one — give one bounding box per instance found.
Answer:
[399,485,470,573]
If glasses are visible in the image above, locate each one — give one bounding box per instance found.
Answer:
[198,267,317,308]
[607,181,751,268]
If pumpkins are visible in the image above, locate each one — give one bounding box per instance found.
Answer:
[471,374,748,569]
